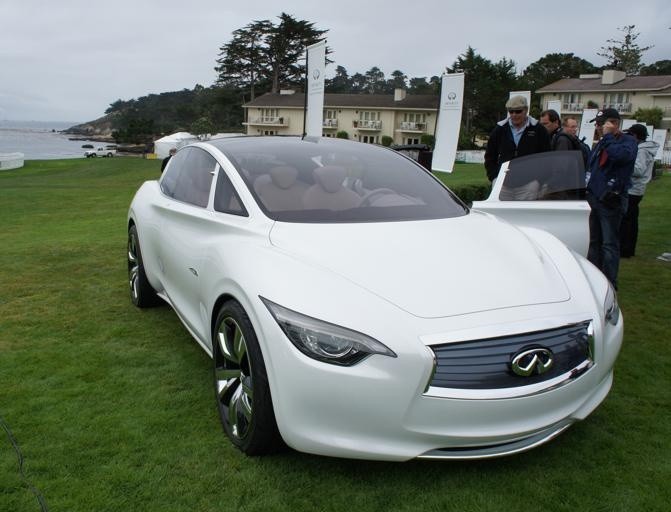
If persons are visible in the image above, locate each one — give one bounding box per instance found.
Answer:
[161,148,177,172]
[587,108,637,292]
[538,109,573,152]
[560,116,592,173]
[484,95,551,184]
[622,124,660,258]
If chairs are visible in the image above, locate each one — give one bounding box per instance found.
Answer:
[300,163,362,211]
[252,163,311,214]
[230,169,263,214]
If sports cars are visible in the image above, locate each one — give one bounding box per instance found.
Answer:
[125,131,628,469]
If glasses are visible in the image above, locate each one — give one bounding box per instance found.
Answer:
[508,109,524,114]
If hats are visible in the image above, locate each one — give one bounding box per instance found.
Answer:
[623,122,650,137]
[504,94,529,109]
[588,108,621,123]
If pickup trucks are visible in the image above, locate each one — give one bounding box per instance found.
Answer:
[83,146,117,159]
